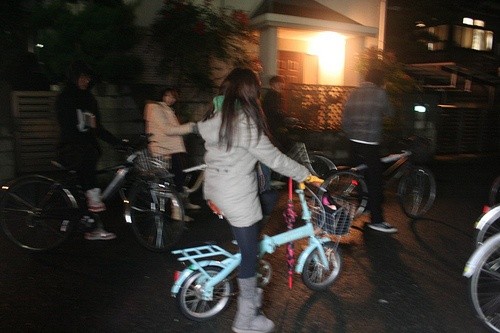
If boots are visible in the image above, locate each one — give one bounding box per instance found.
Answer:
[232,288,275,333]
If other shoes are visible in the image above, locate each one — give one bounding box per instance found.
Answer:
[171,207,194,221]
[184,203,201,209]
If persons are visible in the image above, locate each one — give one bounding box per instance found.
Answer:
[54,64,131,240]
[342,67,399,232]
[144,88,202,222]
[260,73,306,188]
[196,66,325,331]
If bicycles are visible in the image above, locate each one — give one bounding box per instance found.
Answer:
[320,122,442,222]
[0,131,187,257]
[168,139,355,323]
[462,175,500,332]
[253,118,337,198]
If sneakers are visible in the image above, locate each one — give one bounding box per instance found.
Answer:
[85,227,118,240]
[368,221,398,233]
[88,201,106,212]
